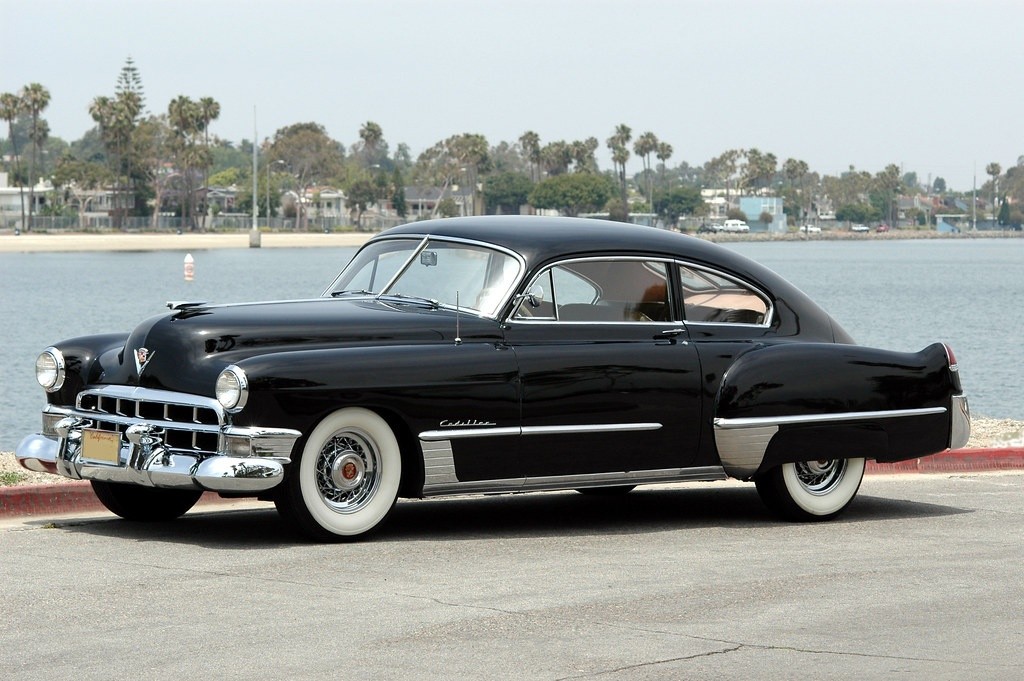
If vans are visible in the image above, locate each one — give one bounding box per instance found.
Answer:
[724,220,750,232]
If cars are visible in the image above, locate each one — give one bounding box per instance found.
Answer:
[698,221,722,234]
[877,224,889,232]
[850,223,869,232]
[799,224,821,234]
[17,213,971,542]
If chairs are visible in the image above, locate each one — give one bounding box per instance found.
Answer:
[554,303,642,321]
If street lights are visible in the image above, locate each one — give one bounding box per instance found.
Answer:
[449,168,467,201]
[29,149,50,218]
[266,159,285,230]
[769,181,784,215]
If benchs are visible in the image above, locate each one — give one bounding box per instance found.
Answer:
[592,298,764,326]
